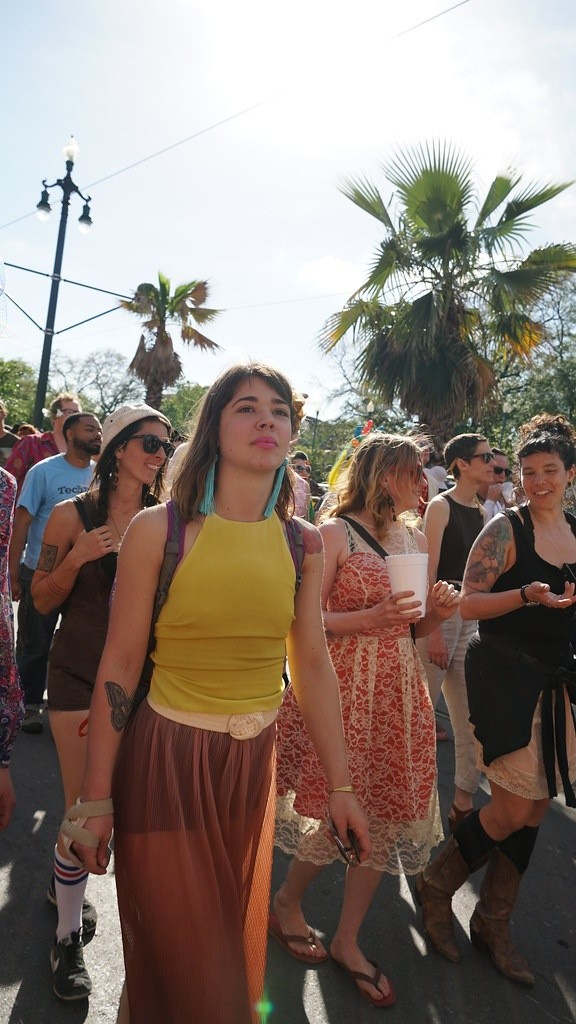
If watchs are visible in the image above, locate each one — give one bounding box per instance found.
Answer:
[520,584,538,606]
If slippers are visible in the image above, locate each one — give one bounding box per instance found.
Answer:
[268,912,327,963]
[332,954,397,1007]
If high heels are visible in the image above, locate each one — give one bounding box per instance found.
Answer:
[448,803,474,832]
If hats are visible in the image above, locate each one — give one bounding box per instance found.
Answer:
[99,401,172,454]
[423,465,448,490]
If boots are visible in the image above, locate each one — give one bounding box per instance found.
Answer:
[414,832,472,961]
[470,848,535,985]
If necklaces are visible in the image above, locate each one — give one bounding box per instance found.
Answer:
[108,509,121,539]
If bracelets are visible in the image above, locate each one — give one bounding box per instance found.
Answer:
[329,786,355,795]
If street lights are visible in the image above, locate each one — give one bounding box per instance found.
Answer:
[34,152,93,426]
[311,406,322,454]
[499,394,513,449]
[367,401,376,420]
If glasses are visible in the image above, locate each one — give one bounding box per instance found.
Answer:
[466,453,495,463]
[327,814,361,867]
[494,466,511,476]
[295,465,312,474]
[58,408,79,416]
[127,435,175,458]
[390,464,421,483]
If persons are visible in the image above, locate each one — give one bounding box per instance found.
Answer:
[8,412,103,736]
[424,433,497,832]
[413,414,576,983]
[60,364,373,1024]
[2,394,83,504]
[410,430,512,536]
[0,466,26,832]
[275,432,461,1007]
[30,400,176,1000]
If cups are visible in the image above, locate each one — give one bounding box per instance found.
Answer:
[386,553,428,618]
[501,481,516,503]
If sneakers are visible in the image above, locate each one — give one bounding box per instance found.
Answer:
[47,925,93,999]
[48,881,97,932]
[22,705,43,732]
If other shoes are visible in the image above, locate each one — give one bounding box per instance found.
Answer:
[436,724,448,741]
